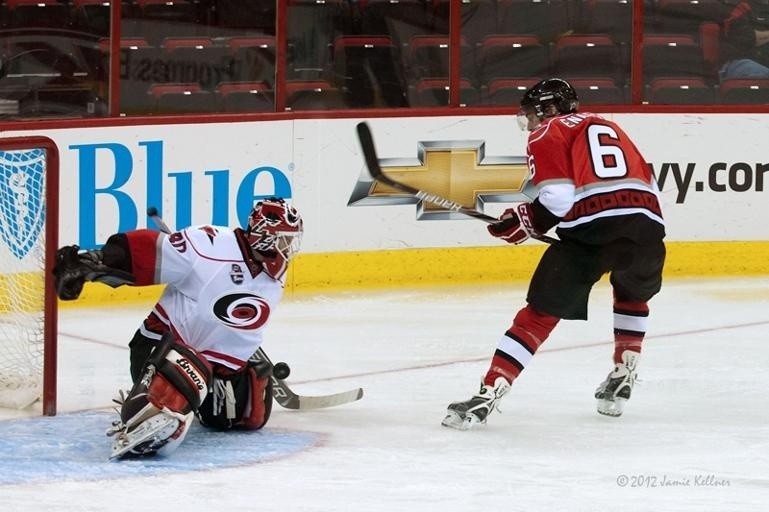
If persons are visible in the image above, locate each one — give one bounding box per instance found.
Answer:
[52,197,303,458]
[644,0,769,87]
[447,79,665,425]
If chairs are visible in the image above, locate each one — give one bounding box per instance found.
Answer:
[92,34,769,114]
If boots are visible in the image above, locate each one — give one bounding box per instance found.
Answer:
[595,350,642,400]
[447,375,510,421]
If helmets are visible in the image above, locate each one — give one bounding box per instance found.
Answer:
[516,79,579,131]
[249,197,303,260]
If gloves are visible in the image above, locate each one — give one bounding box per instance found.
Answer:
[488,204,542,244]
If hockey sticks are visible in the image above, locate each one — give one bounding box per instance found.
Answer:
[150,208,364,411]
[356,122,559,243]
